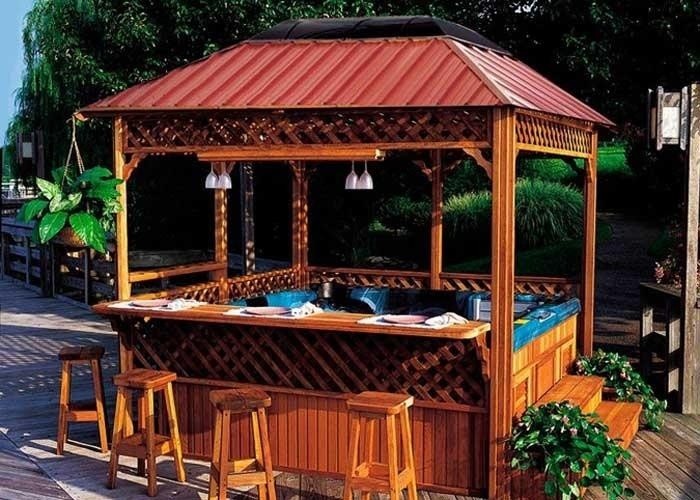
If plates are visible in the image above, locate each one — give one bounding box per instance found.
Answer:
[132,299,173,308]
[245,306,291,315]
[383,314,429,324]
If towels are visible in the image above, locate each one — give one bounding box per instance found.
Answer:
[425,311,470,327]
[166,297,208,310]
[291,300,324,318]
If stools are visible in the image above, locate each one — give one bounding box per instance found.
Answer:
[343,390,418,500]
[107,368,186,496]
[209,388,277,500]
[57,345,109,455]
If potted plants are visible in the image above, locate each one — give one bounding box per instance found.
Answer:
[19,163,121,249]
